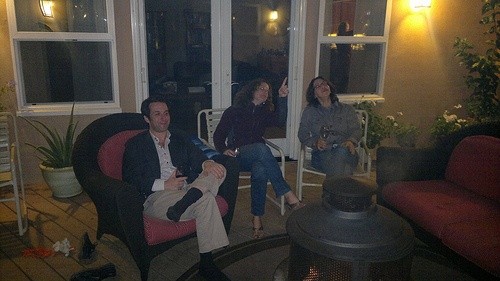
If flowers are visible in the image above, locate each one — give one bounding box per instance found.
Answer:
[353,98,388,148]
[428,110,472,143]
[383,110,419,147]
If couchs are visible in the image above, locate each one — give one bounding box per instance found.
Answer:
[374,119,500,278]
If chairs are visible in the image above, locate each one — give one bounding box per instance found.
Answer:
[296,108,372,200]
[0,112,30,236]
[70,111,240,281]
[197,107,287,216]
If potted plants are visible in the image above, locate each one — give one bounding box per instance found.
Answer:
[15,102,84,200]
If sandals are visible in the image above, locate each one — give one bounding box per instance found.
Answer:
[253,222,264,239]
[288,201,305,210]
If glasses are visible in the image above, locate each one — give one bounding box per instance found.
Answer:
[312,82,327,92]
[256,88,269,94]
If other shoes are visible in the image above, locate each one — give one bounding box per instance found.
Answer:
[78,233,95,258]
[71,264,116,281]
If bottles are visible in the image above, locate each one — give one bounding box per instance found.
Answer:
[316,123,330,151]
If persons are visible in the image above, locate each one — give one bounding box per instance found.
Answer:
[298,75,363,197]
[122,96,231,281]
[213,77,306,241]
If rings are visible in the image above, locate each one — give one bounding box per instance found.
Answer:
[219,166,222,168]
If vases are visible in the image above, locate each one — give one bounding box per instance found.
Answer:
[365,146,378,168]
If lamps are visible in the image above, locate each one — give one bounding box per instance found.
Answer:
[265,7,280,23]
[39,0,57,18]
